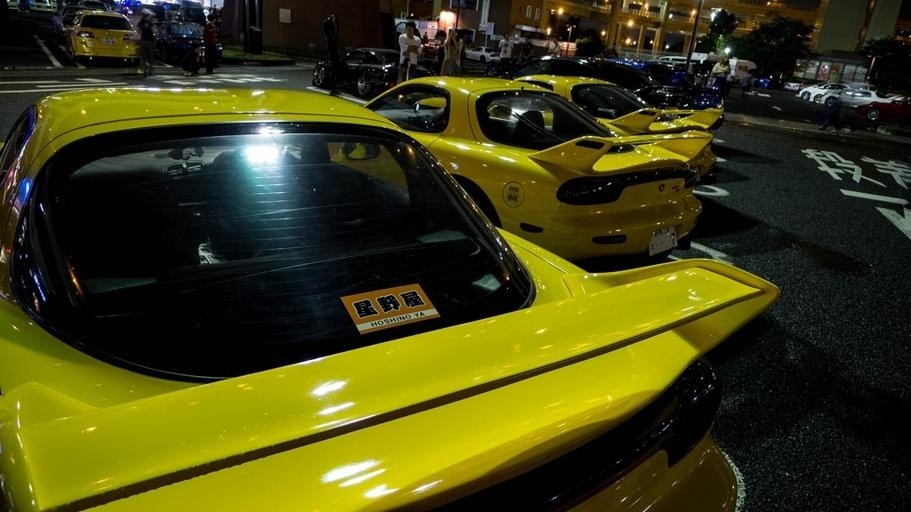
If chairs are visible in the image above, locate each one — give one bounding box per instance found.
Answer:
[202,149,317,262]
[511,110,545,147]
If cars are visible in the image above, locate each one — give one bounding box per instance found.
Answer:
[5,0,211,69]
[2,89,781,506]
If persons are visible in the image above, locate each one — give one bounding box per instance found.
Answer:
[820,72,841,131]
[710,57,731,96]
[547,35,561,57]
[203,13,220,74]
[439,28,463,76]
[741,69,756,101]
[136,13,155,77]
[519,35,534,61]
[396,21,422,86]
[499,31,514,63]
[423,32,428,39]
[181,40,202,77]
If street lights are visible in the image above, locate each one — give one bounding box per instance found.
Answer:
[609,15,636,63]
[553,7,564,39]
[566,24,578,56]
